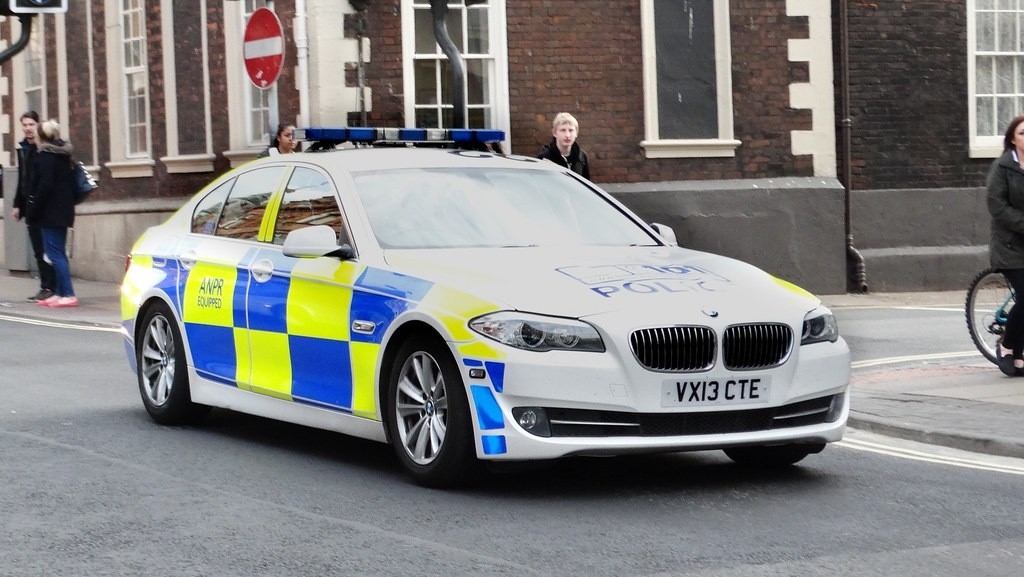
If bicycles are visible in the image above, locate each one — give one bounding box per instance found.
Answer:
[965,267,1024,367]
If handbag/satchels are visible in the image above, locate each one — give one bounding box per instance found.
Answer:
[70,159,98,203]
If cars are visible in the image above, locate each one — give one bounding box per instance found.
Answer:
[119,127,851,489]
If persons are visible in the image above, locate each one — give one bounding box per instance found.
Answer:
[25,119,79,307]
[534,113,590,181]
[12,111,56,303]
[257,123,298,159]
[986,116,1024,378]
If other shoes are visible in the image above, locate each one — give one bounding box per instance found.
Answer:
[48,295,80,308]
[26,288,55,302]
[1013,363,1024,376]
[996,343,1015,376]
[39,300,59,306]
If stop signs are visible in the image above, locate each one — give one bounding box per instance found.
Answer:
[243,8,285,89]
